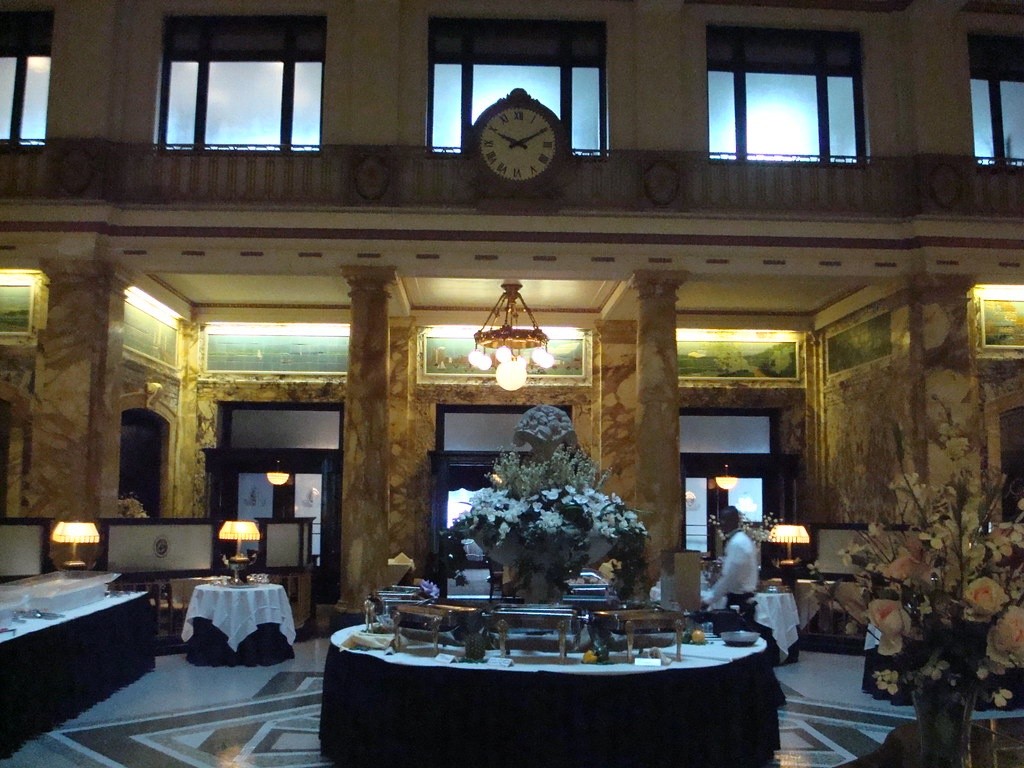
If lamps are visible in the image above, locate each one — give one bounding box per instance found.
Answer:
[768,525,810,565]
[51,522,100,578]
[467,280,555,391]
[218,519,261,588]
[267,472,290,486]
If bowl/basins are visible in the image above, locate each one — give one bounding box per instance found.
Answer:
[720,630,761,647]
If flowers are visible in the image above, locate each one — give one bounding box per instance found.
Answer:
[581,644,608,662]
[680,627,705,643]
[344,636,386,651]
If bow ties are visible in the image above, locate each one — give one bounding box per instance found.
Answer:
[723,530,740,543]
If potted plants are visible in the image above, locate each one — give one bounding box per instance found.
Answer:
[437,443,652,601]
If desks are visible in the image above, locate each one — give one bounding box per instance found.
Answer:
[181,580,297,668]
[318,622,787,768]
[0,591,157,760]
[749,592,800,668]
[833,716,1024,768]
[805,419,1024,708]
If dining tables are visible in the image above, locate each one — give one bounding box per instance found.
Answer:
[911,688,976,768]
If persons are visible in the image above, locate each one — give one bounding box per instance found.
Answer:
[692,506,758,624]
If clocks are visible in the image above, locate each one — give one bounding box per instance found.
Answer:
[479,106,557,180]
